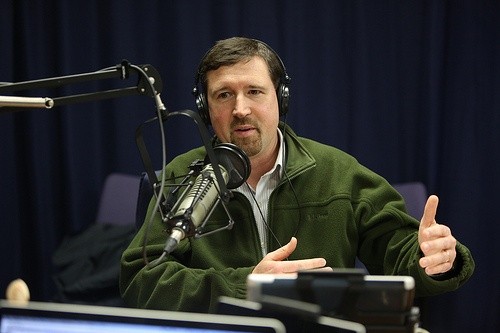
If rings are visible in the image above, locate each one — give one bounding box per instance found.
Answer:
[445,251,450,263]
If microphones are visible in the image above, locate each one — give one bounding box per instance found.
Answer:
[164,162,228,252]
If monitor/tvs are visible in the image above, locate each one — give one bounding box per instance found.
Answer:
[0,300,286,333]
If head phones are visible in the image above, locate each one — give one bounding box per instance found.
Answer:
[194,38,289,126]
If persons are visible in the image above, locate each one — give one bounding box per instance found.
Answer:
[118,36,476,315]
